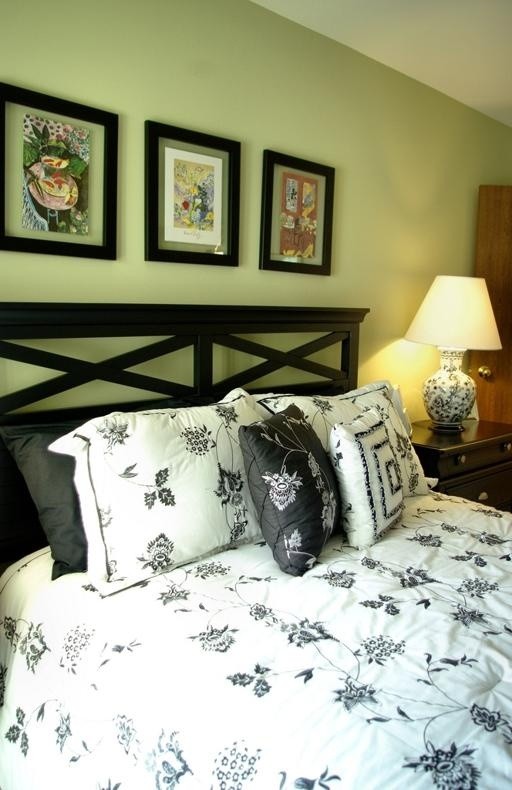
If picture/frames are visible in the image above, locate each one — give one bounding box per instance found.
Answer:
[260,149,335,275]
[144,120,239,267]
[0,82,118,261]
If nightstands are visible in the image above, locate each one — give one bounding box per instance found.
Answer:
[409,419,512,512]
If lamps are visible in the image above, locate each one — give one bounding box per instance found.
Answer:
[405,275,503,434]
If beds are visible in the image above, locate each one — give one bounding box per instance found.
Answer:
[1,301,511,790]
